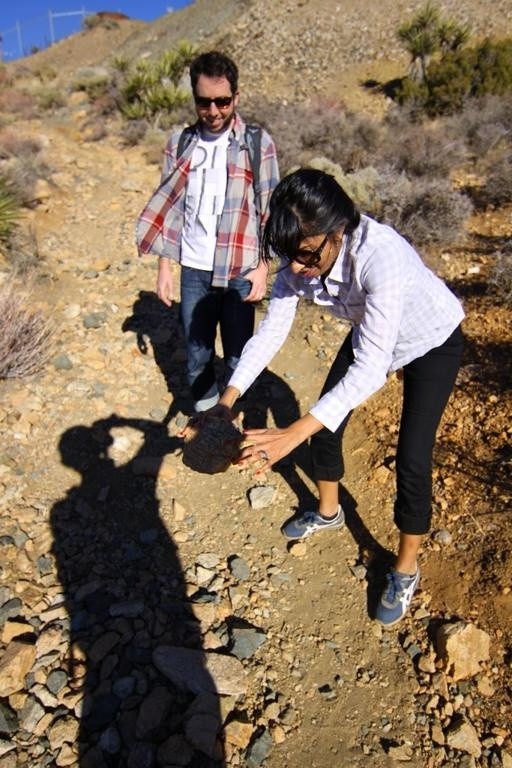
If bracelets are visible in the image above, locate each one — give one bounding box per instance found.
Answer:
[156,258,174,266]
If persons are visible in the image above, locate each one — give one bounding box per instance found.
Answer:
[136,46,281,416]
[176,166,466,626]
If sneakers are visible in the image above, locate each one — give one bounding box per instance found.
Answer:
[374,561,420,626]
[280,502,345,541]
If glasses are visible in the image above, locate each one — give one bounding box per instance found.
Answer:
[283,231,332,263]
[194,92,235,108]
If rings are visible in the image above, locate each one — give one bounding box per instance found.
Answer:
[259,449,269,459]
